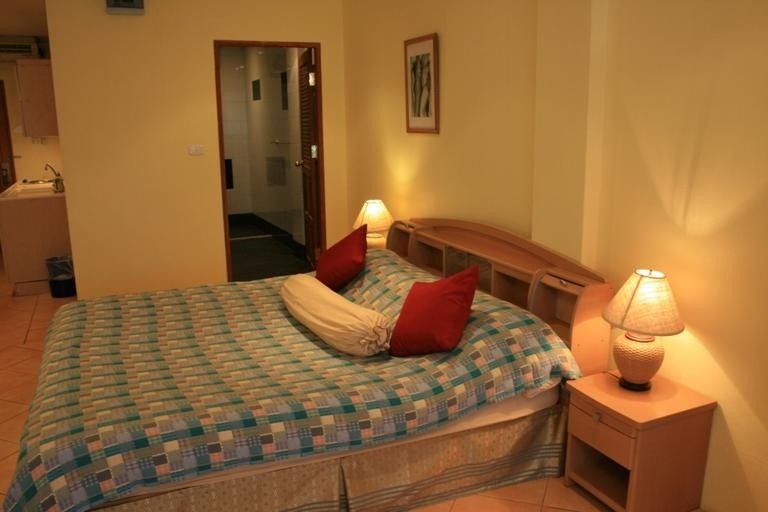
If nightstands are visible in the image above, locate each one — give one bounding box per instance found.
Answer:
[564,369,719,512]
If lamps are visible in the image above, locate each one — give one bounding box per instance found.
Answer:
[601,267,684,390]
[352,197,394,248]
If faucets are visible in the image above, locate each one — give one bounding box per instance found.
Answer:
[44,163,57,176]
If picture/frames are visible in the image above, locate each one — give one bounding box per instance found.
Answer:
[404,33,441,134]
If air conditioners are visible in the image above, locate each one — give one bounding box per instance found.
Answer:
[0,36,39,63]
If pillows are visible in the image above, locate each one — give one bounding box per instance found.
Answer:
[280,273,392,356]
[388,263,478,357]
[316,224,367,290]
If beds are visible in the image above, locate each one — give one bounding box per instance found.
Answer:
[1,218,614,512]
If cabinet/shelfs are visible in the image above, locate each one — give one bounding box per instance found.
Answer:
[15,59,58,138]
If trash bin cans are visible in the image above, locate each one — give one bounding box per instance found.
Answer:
[46,255,75,298]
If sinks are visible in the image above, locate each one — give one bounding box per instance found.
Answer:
[21,187,52,193]
